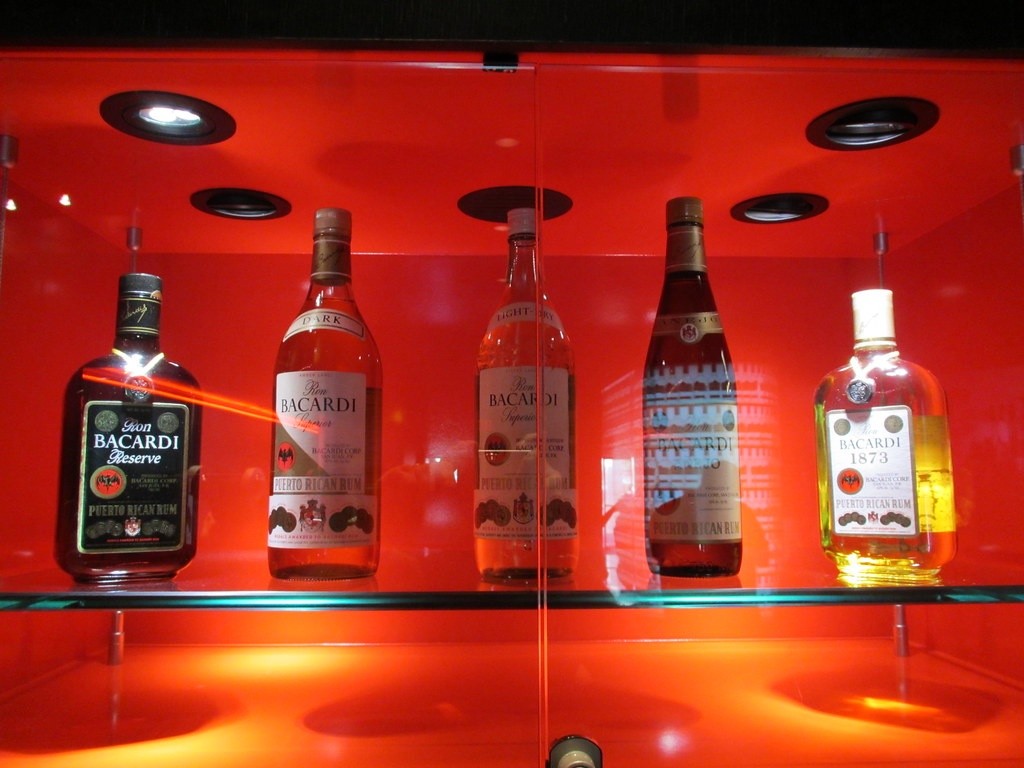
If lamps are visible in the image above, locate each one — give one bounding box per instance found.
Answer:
[210,192,274,217]
[825,107,917,145]
[744,199,815,222]
[137,106,201,127]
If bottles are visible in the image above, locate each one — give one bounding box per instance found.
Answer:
[642,197,745,575]
[268,207,382,579]
[54,273,203,583]
[813,289,957,582]
[473,208,579,581]
